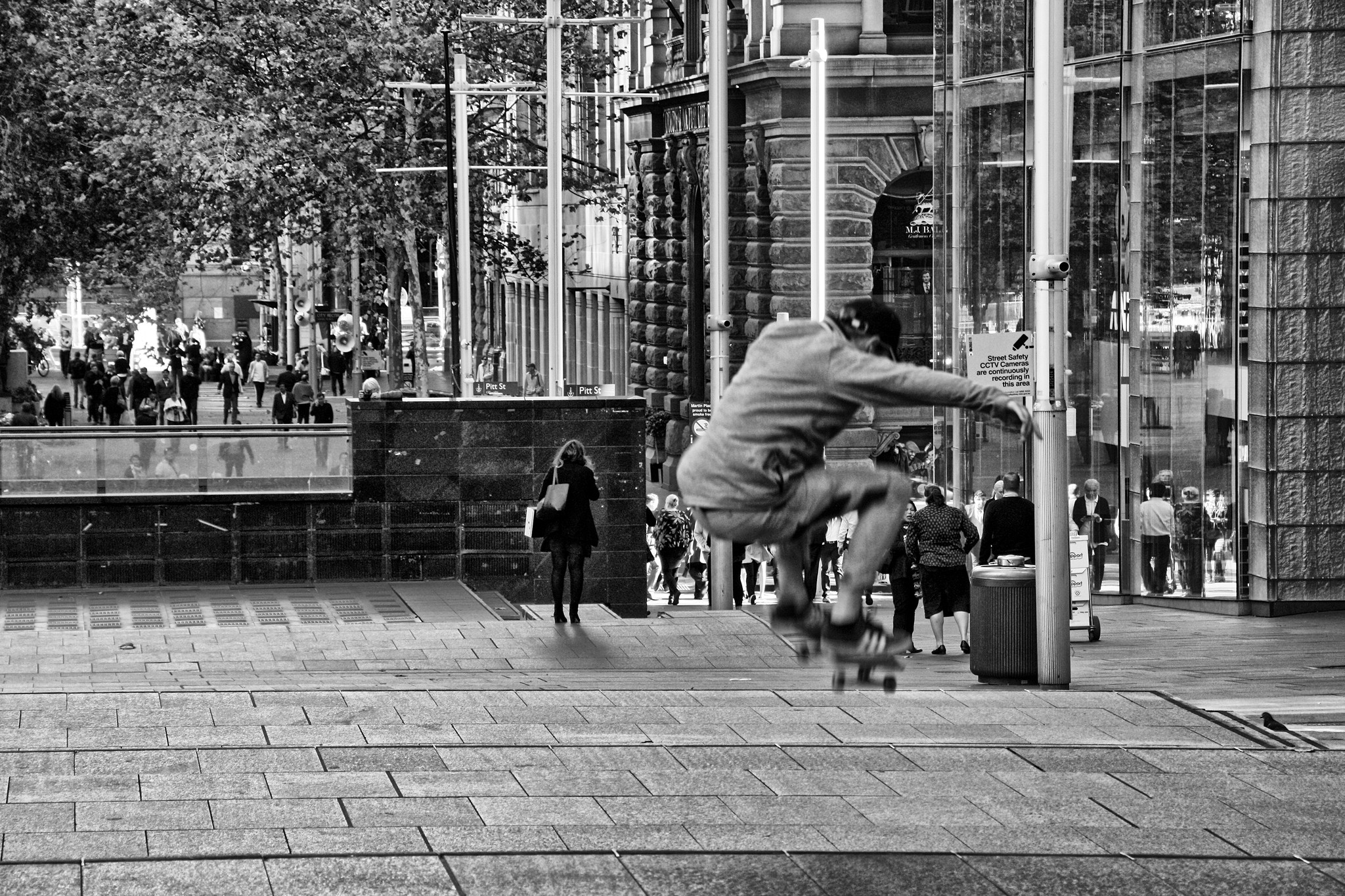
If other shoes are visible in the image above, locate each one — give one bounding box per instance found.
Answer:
[340,389,346,395]
[1207,574,1212,582]
[73,405,77,408]
[748,594,756,605]
[100,421,106,425]
[654,580,681,605]
[80,404,84,410]
[960,640,970,654]
[87,416,92,422]
[931,645,946,654]
[92,422,97,425]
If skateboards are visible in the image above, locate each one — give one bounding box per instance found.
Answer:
[767,603,906,697]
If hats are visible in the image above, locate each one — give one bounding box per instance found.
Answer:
[841,300,900,362]
[526,363,535,367]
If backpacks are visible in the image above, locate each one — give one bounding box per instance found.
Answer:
[1176,504,1199,539]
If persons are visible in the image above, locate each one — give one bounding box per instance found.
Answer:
[540,440,600,625]
[889,498,923,658]
[979,473,1035,565]
[523,363,544,397]
[1068,470,1211,597]
[328,348,347,396]
[218,421,255,478]
[268,344,328,396]
[292,374,315,431]
[410,341,429,388]
[217,363,242,424]
[915,481,1006,580]
[1173,325,1201,379]
[310,393,334,469]
[906,484,979,655]
[247,353,269,408]
[216,327,252,415]
[476,355,493,382]
[329,451,349,475]
[358,378,403,400]
[10,316,223,481]
[272,383,299,449]
[677,297,1044,663]
[645,492,875,608]
[915,270,933,335]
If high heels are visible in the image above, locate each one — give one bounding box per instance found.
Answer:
[902,641,922,655]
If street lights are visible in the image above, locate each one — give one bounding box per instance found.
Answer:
[461,1,648,397]
[384,51,537,397]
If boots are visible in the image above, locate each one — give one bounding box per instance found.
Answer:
[554,603,567,624]
[569,602,580,624]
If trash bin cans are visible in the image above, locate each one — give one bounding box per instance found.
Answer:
[968,553,1036,687]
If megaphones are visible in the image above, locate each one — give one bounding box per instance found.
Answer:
[337,313,354,332]
[294,311,310,327]
[335,334,355,353]
[294,296,311,312]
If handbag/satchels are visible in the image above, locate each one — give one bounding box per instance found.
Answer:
[542,466,569,510]
[184,414,190,422]
[119,410,135,426]
[293,405,297,418]
[117,387,127,407]
[321,367,329,375]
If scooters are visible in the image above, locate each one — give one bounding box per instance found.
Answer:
[27,340,49,377]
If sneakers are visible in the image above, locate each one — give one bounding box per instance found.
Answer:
[769,593,831,637]
[819,611,912,660]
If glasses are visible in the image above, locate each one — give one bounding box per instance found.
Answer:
[482,359,486,360]
